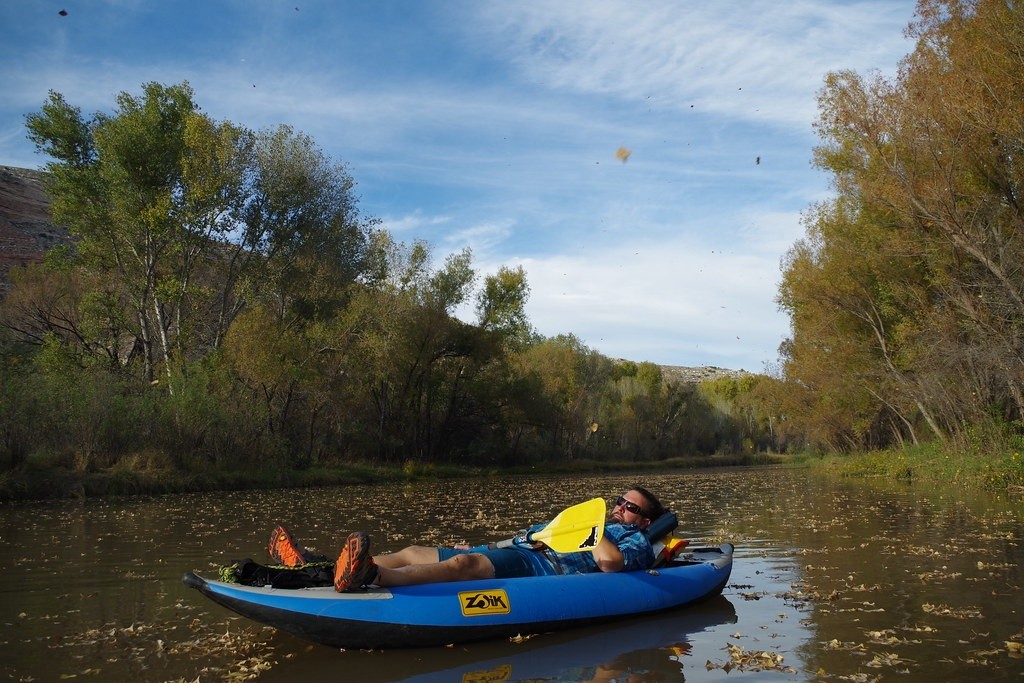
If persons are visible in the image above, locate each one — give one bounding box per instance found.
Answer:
[269,487,662,595]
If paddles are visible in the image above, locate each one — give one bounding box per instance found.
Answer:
[468,495,608,556]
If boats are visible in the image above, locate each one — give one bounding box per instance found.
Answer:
[179,542,739,649]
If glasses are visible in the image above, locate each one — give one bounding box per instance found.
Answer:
[617,496,648,518]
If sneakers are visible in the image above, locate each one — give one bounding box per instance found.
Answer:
[268,524,306,567]
[333,532,376,593]
